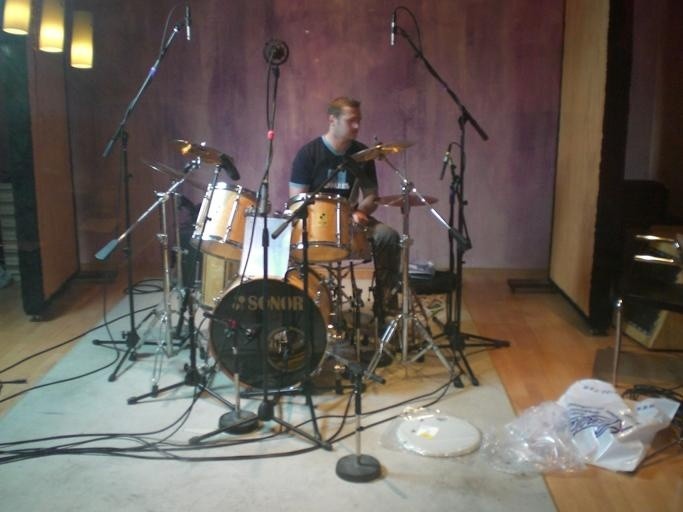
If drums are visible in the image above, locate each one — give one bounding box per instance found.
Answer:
[209,263,334,393]
[190,182,261,264]
[284,192,372,269]
[199,254,241,310]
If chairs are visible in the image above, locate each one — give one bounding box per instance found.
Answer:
[610,177,683,385]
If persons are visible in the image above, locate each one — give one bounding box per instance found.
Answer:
[287,96,402,326]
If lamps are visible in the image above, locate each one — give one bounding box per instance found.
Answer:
[1,0,34,37]
[68,0,95,69]
[37,0,67,53]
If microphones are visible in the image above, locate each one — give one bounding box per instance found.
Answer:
[389,12,396,46]
[185,4,191,40]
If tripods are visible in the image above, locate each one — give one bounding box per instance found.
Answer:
[393,115,510,388]
[129,199,210,401]
[242,205,357,439]
[302,257,387,416]
[125,286,236,412]
[162,185,213,353]
[186,166,334,453]
[93,130,183,382]
[361,182,466,388]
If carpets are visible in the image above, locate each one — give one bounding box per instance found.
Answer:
[0,275,558,512]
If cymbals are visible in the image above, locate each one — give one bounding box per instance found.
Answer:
[373,194,439,207]
[168,139,234,164]
[349,140,416,163]
[139,158,207,191]
